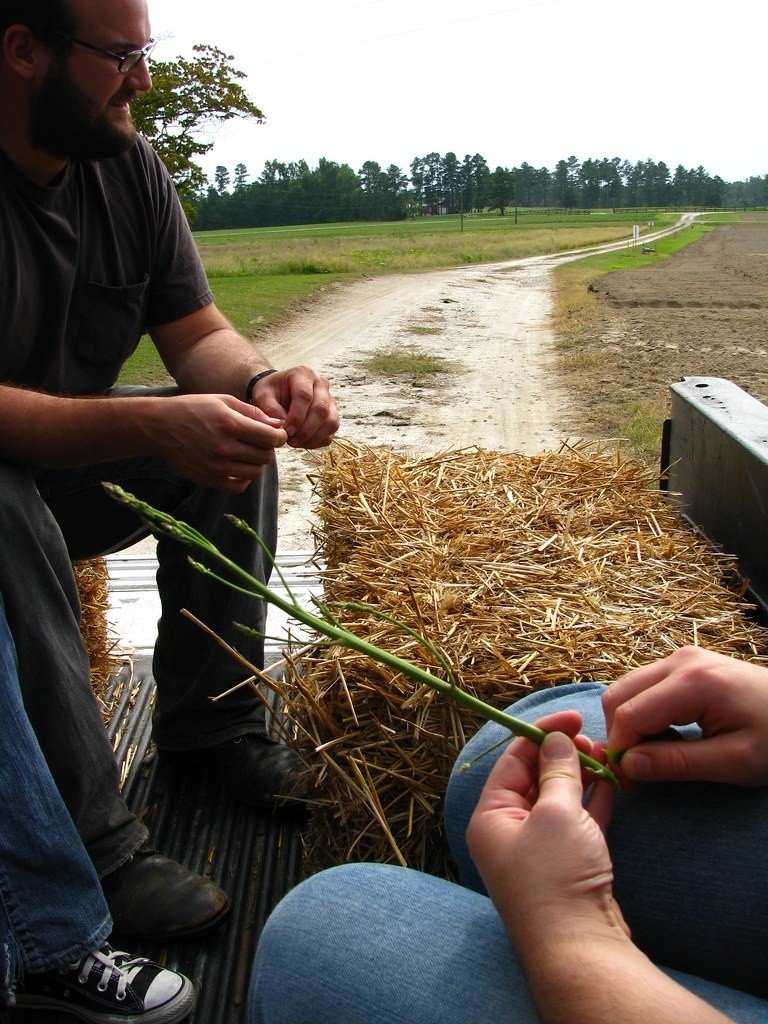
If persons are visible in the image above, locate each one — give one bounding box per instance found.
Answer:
[0,601,198,1024]
[244,645,768,1024]
[0,0,343,936]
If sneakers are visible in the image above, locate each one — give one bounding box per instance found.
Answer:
[11,942,195,1024]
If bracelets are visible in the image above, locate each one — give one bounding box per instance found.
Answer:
[246,368,278,404]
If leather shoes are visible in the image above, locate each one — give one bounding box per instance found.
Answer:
[158,733,314,808]
[101,839,232,939]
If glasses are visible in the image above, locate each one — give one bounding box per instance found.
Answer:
[67,38,157,74]
